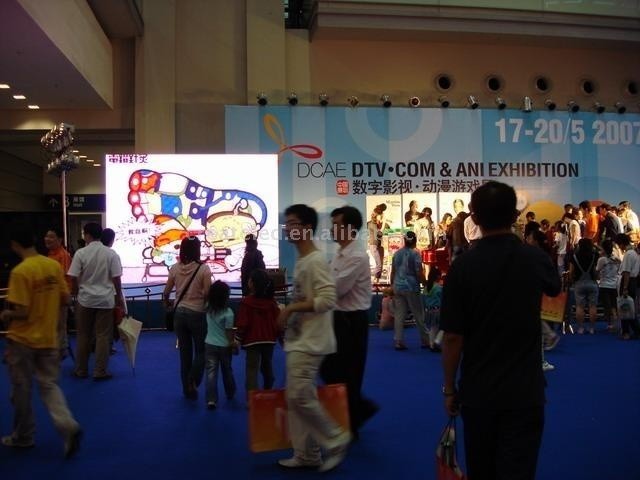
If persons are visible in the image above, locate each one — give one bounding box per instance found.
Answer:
[101,227,116,247]
[574,206,587,237]
[374,204,390,221]
[596,242,620,330]
[441,212,452,229]
[571,238,597,334]
[163,235,210,406]
[201,280,237,406]
[321,206,381,433]
[367,212,385,282]
[424,267,446,350]
[278,204,353,470]
[239,269,278,391]
[444,179,559,480]
[578,200,599,240]
[525,211,539,237]
[546,224,555,249]
[44,227,73,358]
[241,233,268,296]
[421,206,436,229]
[427,231,454,348]
[0,222,84,461]
[404,200,421,226]
[389,231,433,349]
[598,202,623,241]
[616,231,639,341]
[610,206,619,217]
[563,204,573,215]
[67,221,125,381]
[552,219,568,273]
[618,201,640,236]
[447,210,473,261]
[537,218,549,230]
[563,213,581,250]
[527,230,546,248]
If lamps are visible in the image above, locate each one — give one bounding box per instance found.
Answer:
[439,95,449,108]
[614,101,627,114]
[468,95,478,109]
[544,98,556,110]
[256,91,267,107]
[40,122,102,253]
[568,100,580,112]
[348,96,358,107]
[409,96,420,108]
[495,96,506,110]
[522,96,532,112]
[593,102,605,115]
[381,94,392,107]
[318,92,329,106]
[285,92,297,106]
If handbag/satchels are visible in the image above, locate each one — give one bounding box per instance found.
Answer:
[248,383,353,455]
[436,416,465,479]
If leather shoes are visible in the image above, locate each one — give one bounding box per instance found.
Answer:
[394,340,408,349]
[395,344,408,350]
[278,458,322,467]
[68,370,87,378]
[319,445,351,473]
[427,342,440,351]
[420,340,430,346]
[92,373,113,382]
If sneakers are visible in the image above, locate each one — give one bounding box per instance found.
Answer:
[64,427,84,462]
[1,436,35,449]
[542,361,555,371]
[543,334,561,350]
[208,401,217,411]
